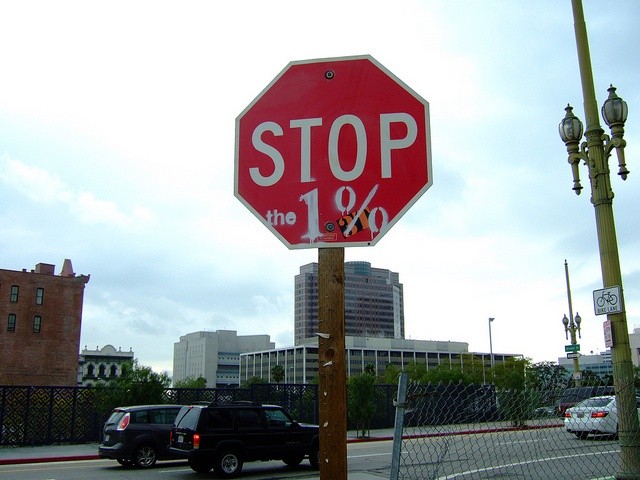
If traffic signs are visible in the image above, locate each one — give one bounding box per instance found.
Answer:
[565,344,580,352]
[567,352,582,359]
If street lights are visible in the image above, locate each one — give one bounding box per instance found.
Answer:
[563,258,582,389]
[556,0,639,480]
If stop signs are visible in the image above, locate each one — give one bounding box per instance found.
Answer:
[233,54,433,250]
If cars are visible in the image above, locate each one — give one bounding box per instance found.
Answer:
[564,395,640,441]
[535,406,553,416]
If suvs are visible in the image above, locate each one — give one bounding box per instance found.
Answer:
[557,386,614,419]
[98,404,188,468]
[168,400,319,479]
